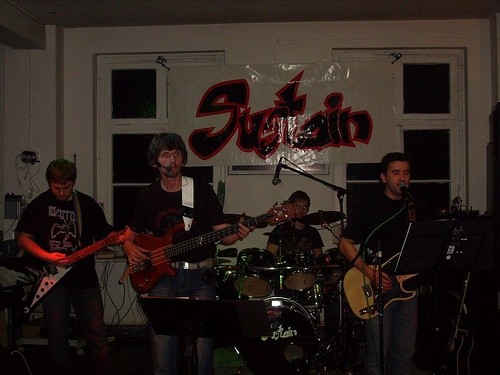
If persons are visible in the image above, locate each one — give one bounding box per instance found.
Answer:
[338,152,422,375]
[123,132,255,375]
[266,190,324,300]
[15,159,127,375]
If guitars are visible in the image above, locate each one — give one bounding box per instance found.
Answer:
[1,228,129,319]
[344,247,500,321]
[125,202,298,296]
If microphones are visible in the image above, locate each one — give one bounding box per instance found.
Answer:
[399,182,409,203]
[160,165,172,171]
[272,157,282,185]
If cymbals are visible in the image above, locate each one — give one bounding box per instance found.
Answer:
[300,208,347,225]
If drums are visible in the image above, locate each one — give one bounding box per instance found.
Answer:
[212,247,353,375]
